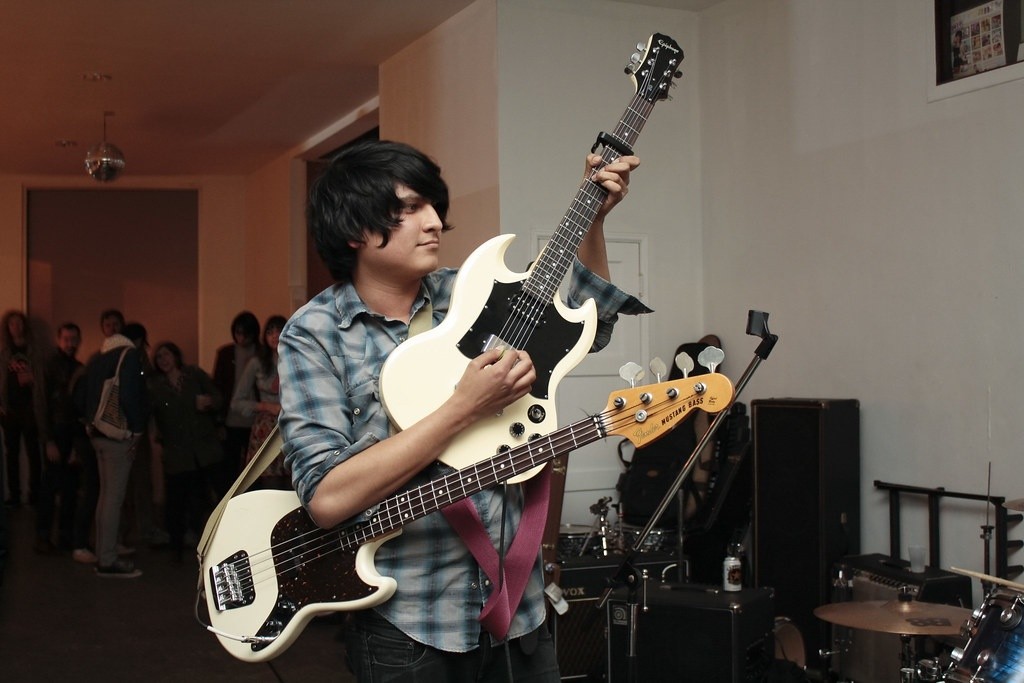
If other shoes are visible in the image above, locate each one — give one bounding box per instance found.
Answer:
[116,544,135,557]
[4,495,22,508]
[33,538,57,555]
[72,548,94,563]
[61,541,93,554]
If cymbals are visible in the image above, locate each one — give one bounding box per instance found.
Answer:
[814,600,983,639]
[1000,498,1024,512]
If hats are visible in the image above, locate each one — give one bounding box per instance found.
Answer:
[123,323,151,347]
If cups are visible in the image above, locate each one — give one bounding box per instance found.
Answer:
[196,395,206,410]
[908,545,926,573]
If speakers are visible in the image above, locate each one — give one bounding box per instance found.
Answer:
[549,397,974,683]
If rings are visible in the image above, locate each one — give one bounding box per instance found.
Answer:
[132,447,135,450]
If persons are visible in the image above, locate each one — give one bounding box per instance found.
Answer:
[277,138,640,683]
[33,310,286,579]
[1,308,44,513]
[952,16,1003,73]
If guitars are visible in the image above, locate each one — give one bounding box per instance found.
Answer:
[192,345,736,665]
[377,27,688,484]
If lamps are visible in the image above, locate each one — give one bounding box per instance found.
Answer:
[84,110,127,183]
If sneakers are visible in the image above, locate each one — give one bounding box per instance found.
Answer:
[94,559,143,578]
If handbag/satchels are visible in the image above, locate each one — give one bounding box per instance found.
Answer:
[93,347,134,440]
[248,410,286,476]
